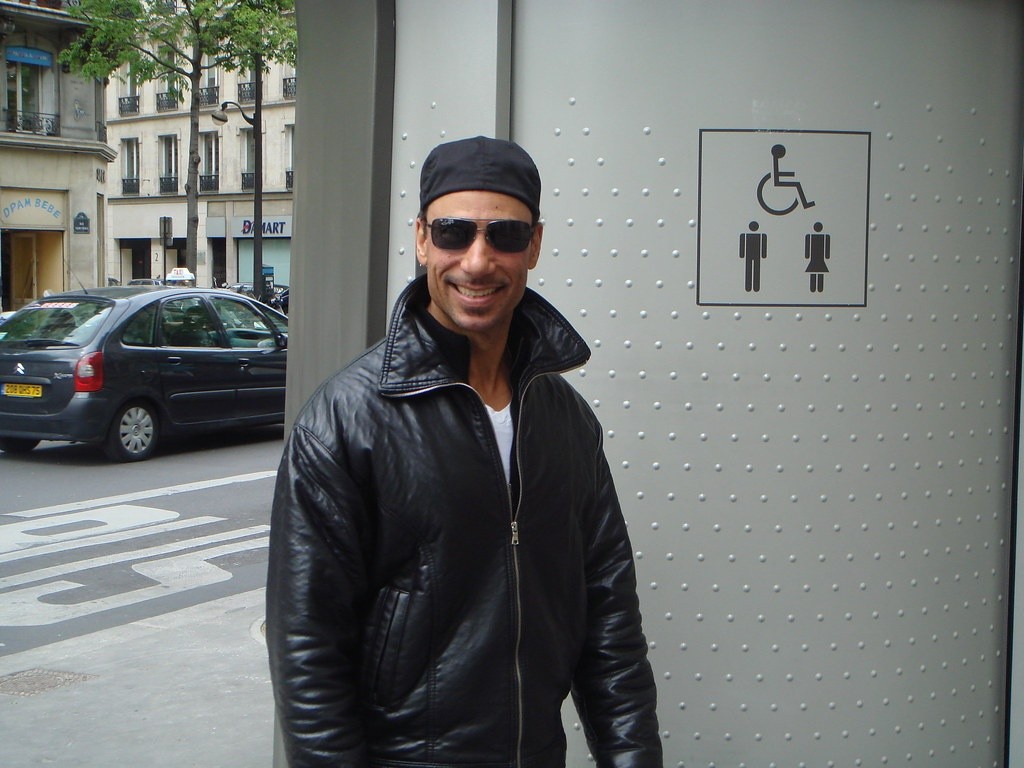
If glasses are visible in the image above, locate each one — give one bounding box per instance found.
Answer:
[425,218,535,253]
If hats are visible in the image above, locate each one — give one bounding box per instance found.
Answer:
[420,137,541,226]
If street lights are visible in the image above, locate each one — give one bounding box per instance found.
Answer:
[211,100,269,305]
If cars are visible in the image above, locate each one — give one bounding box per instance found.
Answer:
[0,285,288,462]
[226,292,289,345]
[266,284,290,313]
[124,278,187,290]
[107,276,121,289]
[228,282,289,303]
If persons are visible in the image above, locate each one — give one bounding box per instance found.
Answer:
[265,135,664,768]
[171,307,215,346]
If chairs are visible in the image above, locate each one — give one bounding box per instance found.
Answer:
[173,305,214,349]
